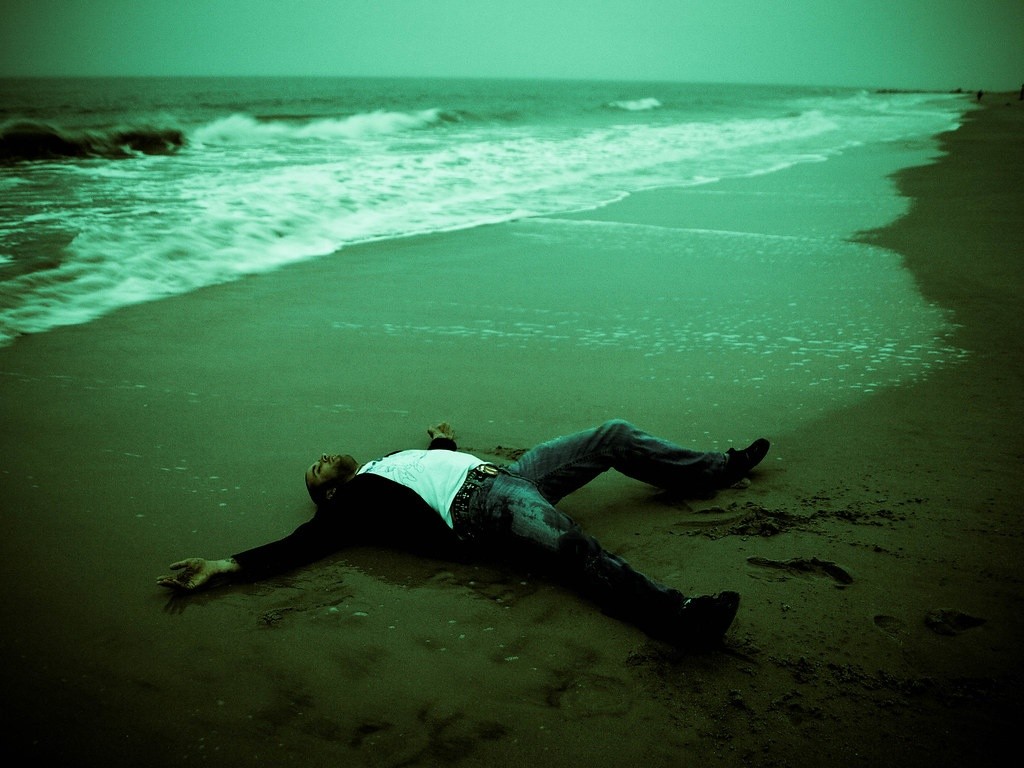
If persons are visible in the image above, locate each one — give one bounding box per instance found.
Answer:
[156,422,772,653]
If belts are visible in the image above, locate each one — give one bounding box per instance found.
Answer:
[454,464,500,545]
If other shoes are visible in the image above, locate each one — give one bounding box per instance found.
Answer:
[717,438,770,490]
[679,591,741,656]
